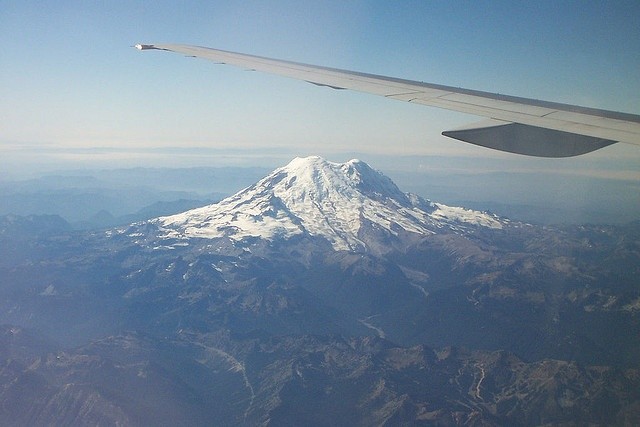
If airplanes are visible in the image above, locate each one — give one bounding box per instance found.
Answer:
[132,41,639,159]
